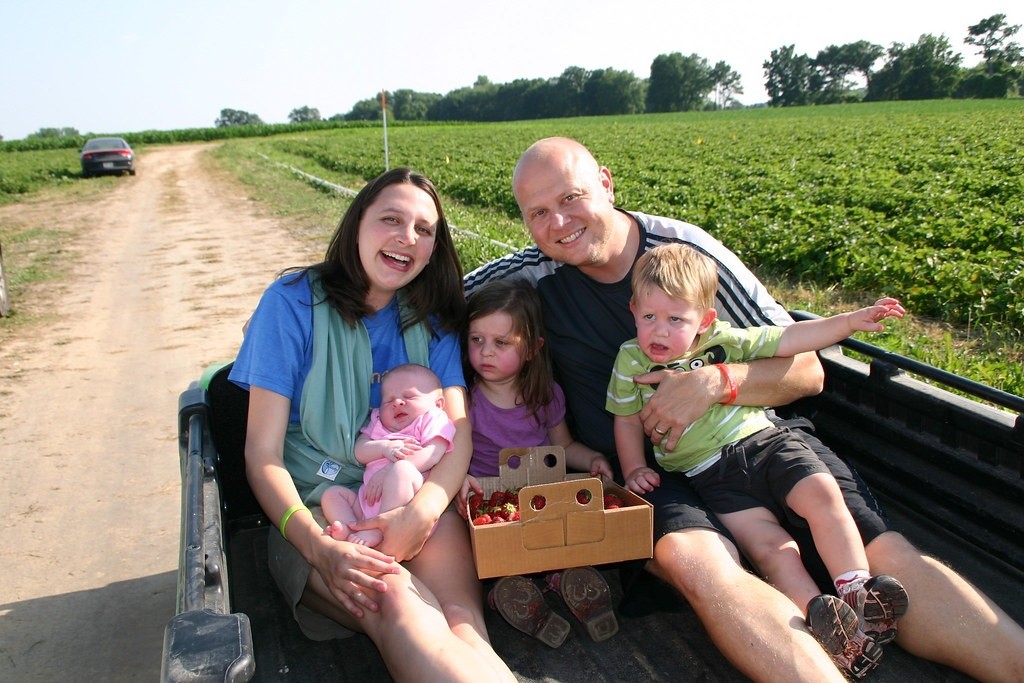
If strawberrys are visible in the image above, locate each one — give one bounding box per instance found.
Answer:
[469,486,625,525]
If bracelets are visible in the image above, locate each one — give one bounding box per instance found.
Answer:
[714,363,736,407]
[279,504,312,541]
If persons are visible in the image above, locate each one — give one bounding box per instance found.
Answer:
[463,137,1024,683]
[454,277,619,648]
[227,168,520,683]
[319,364,457,548]
[605,242,910,683]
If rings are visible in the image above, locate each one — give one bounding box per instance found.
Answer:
[655,427,668,436]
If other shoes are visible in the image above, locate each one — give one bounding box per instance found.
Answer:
[544,566,619,643]
[486,575,571,649]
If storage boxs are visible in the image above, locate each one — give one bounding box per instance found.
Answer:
[465,446,655,579]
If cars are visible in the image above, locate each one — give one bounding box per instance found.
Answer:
[78,137,136,179]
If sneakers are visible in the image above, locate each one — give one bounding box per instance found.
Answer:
[803,574,909,681]
